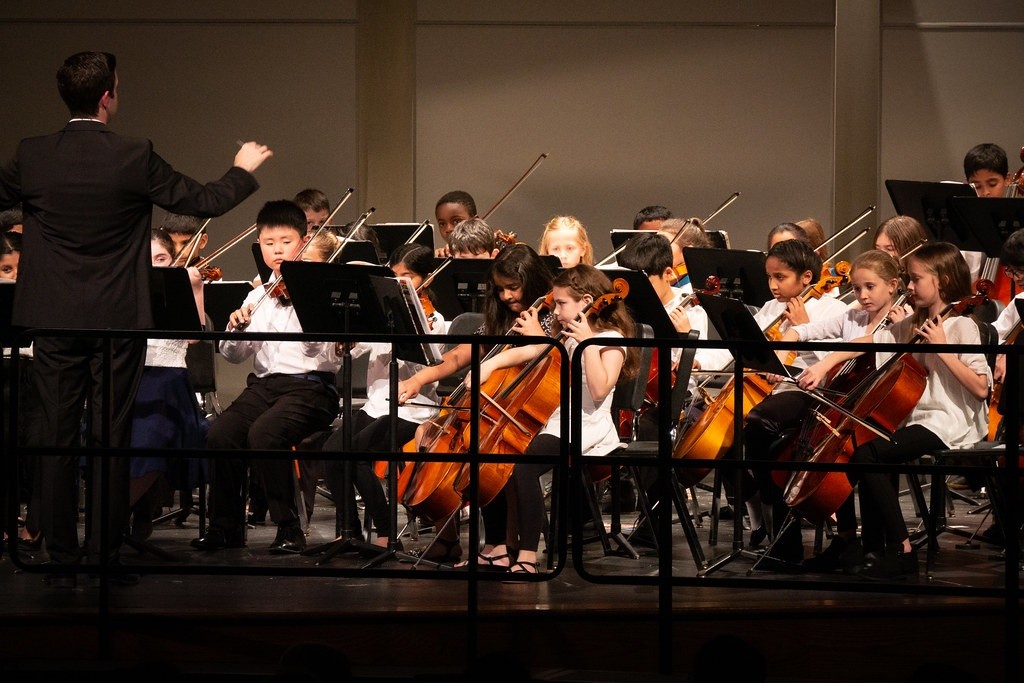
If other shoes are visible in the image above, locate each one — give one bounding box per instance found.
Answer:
[43,569,77,586]
[605,539,660,557]
[800,533,863,575]
[749,512,770,545]
[87,564,143,587]
[752,544,804,574]
[372,541,404,558]
[399,538,463,564]
[858,543,920,585]
[718,505,748,520]
[312,536,365,557]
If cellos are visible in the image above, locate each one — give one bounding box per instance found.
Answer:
[568,276,720,484]
[979,145,1024,307]
[626,261,852,541]
[967,317,1024,545]
[411,278,630,570]
[745,279,996,577]
[772,289,913,490]
[397,291,554,540]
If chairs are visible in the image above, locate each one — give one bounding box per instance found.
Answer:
[185,342,1023,583]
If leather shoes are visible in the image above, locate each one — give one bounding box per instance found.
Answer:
[269,527,307,554]
[191,529,246,549]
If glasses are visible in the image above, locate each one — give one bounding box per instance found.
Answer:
[1004,268,1024,279]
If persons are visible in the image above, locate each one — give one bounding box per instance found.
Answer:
[453,264,641,583]
[190,200,343,554]
[964,143,1011,197]
[246,190,1024,563]
[0,208,222,556]
[796,241,995,581]
[0,50,275,591]
[320,243,444,557]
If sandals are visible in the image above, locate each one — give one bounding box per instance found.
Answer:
[476,553,542,584]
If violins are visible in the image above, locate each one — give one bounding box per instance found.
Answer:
[186,256,223,284]
[820,263,831,278]
[493,229,517,244]
[265,280,290,300]
[418,290,437,331]
